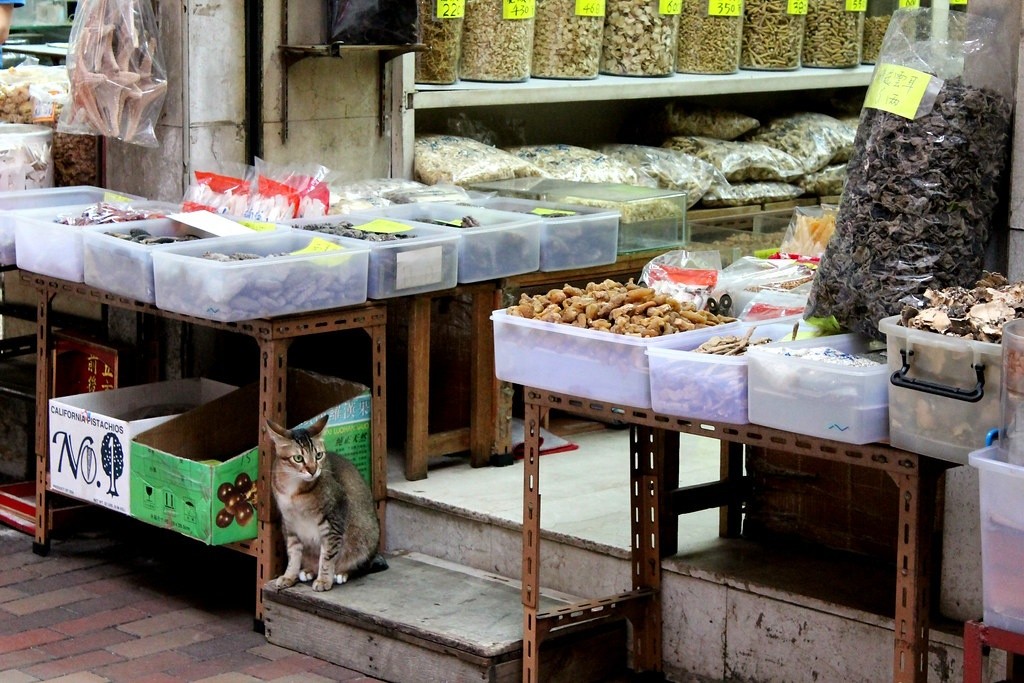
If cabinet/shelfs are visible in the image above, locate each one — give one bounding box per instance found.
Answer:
[391,51,877,181]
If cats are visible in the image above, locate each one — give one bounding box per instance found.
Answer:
[262,414,389,592]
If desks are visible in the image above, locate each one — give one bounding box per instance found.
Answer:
[524,387,937,683]
[17,266,392,633]
[399,207,764,481]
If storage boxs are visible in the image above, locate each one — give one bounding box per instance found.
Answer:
[48,363,373,545]
[1,173,1023,633]
